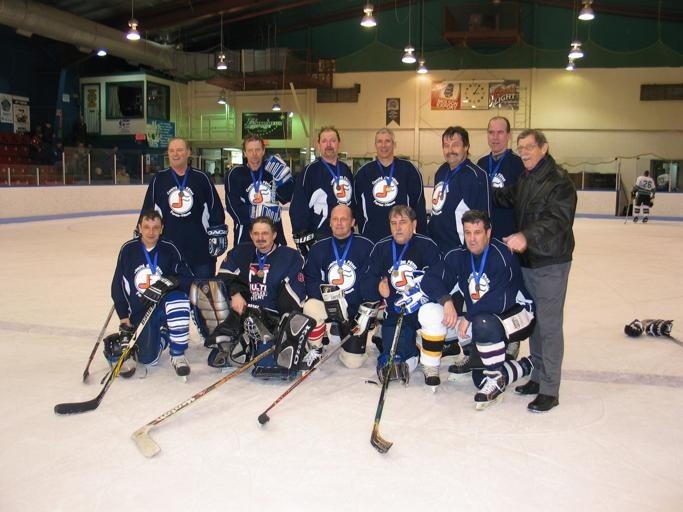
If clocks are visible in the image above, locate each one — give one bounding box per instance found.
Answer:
[461,82,490,109]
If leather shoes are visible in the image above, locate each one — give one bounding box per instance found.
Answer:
[527,394,558,411]
[516,381,539,394]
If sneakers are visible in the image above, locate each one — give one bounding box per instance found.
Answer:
[170,355,189,375]
[299,348,322,370]
[422,339,505,401]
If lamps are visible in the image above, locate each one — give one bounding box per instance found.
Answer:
[217,84,227,104]
[566,0,584,70]
[126,0,141,41]
[272,82,281,111]
[416,0,428,73]
[217,11,228,70]
[360,0,377,27]
[578,0,595,21]
[401,0,416,64]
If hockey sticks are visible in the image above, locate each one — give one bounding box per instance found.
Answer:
[134,344,276,454]
[83,305,115,381]
[56,304,156,413]
[371,305,406,453]
[258,328,360,428]
[623,192,633,224]
[660,319,683,347]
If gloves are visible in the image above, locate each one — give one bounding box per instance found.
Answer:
[350,301,382,337]
[207,224,228,256]
[394,288,430,315]
[292,233,317,256]
[139,278,174,308]
[255,203,281,222]
[645,320,673,335]
[320,284,349,323]
[265,155,292,186]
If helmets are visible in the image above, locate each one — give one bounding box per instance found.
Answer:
[102,334,138,378]
[241,307,275,343]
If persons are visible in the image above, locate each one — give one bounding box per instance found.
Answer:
[493,130,577,412]
[134,138,229,348]
[430,209,537,410]
[208,125,378,383]
[477,116,525,361]
[32,121,130,184]
[359,205,445,391]
[656,167,672,192]
[351,128,427,246]
[631,169,656,222]
[189,148,224,184]
[425,126,492,375]
[111,211,193,384]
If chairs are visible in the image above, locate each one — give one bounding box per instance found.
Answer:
[0,133,74,184]
